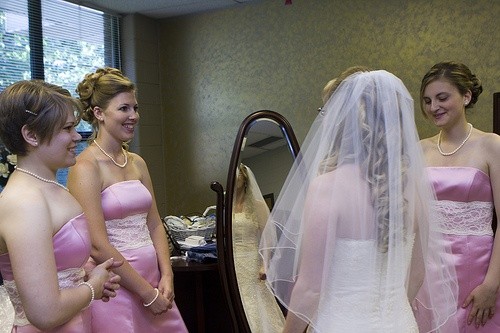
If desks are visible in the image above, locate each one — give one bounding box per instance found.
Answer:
[172,260,217,333]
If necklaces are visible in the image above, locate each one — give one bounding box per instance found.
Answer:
[438,122,472,156]
[14,164,69,191]
[93,138,127,168]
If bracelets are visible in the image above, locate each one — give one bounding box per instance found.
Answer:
[76,282,95,311]
[142,287,159,307]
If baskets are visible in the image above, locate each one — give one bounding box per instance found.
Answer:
[164,205,217,242]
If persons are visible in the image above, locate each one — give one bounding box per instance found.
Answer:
[0,79,124,333]
[67,67,188,333]
[417,62,500,333]
[231,162,286,333]
[259,66,459,333]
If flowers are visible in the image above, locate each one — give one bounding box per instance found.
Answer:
[0,153,20,178]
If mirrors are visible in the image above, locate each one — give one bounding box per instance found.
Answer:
[211,110,307,333]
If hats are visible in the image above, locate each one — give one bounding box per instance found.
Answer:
[177,236,208,248]
